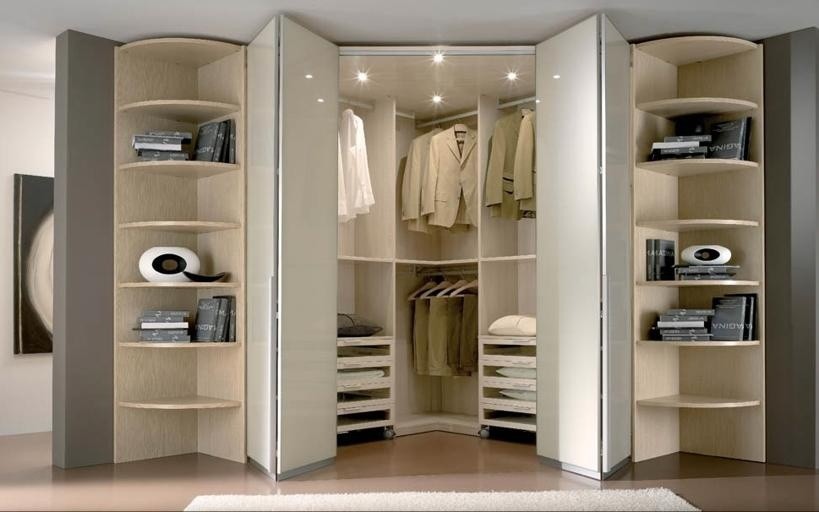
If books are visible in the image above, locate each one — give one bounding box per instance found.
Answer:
[131,119,236,164]
[648,116,752,161]
[133,295,236,342]
[646,239,741,280]
[650,294,757,341]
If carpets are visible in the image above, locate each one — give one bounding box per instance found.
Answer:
[185,488,701,512]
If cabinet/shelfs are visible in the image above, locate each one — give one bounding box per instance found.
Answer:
[600,14,768,482]
[278,13,601,482]
[52,18,276,481]
